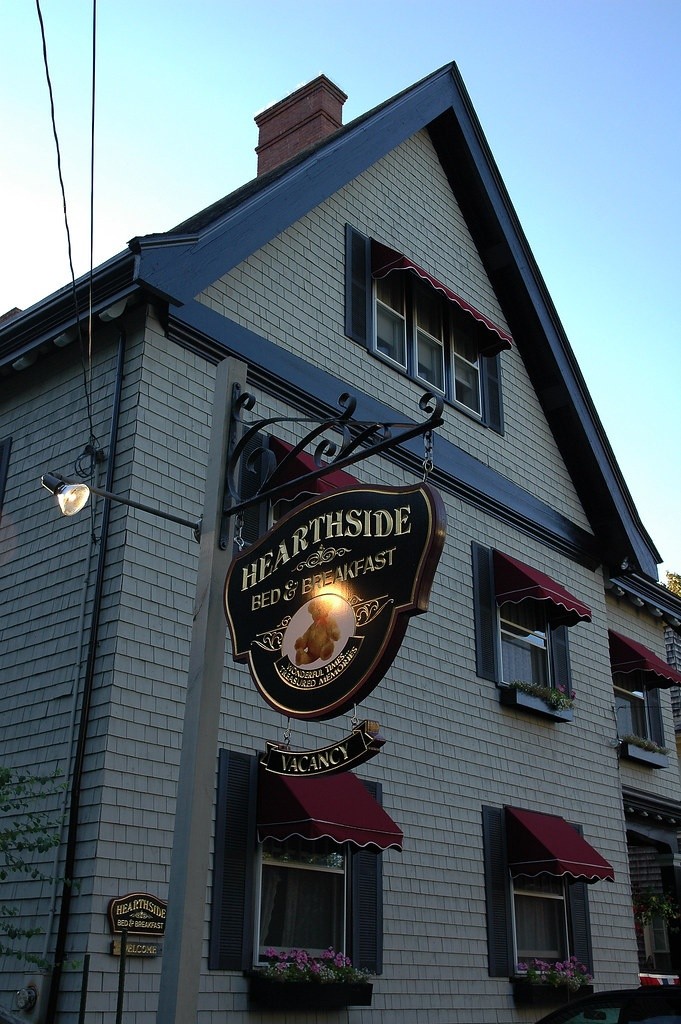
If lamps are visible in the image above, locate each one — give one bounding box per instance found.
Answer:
[40,471,204,544]
[621,558,634,572]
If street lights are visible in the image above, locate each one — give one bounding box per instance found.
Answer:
[42,357,446,1024]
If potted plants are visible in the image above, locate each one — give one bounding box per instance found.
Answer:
[621,733,670,770]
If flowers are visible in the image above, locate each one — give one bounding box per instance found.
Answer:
[263,947,373,987]
[508,678,576,712]
[305,850,344,871]
[515,956,594,992]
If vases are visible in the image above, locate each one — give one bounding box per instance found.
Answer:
[512,984,594,1009]
[502,689,574,723]
[252,982,373,1006]
